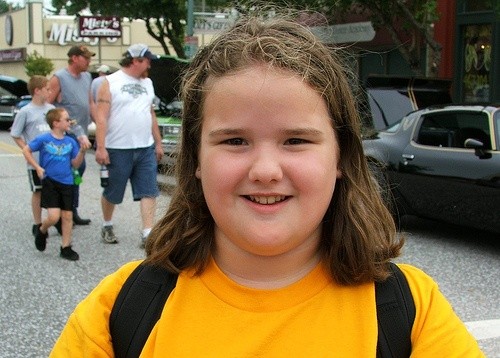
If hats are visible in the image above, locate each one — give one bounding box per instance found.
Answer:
[96,65,110,73]
[67,45,96,60]
[122,43,161,60]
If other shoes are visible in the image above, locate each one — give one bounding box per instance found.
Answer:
[33,223,41,236]
[72,208,91,225]
[101,225,119,243]
[60,244,79,261]
[34,222,49,251]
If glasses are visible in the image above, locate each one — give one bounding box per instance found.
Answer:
[58,118,72,123]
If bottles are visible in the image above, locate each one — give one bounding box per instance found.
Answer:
[72,168,82,185]
[100,164,109,187]
[70,120,90,148]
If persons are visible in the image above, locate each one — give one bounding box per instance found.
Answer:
[45,45,111,226]
[95,43,164,245]
[10,76,63,237]
[48,0,487,358]
[22,108,90,261]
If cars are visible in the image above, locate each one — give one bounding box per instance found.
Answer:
[358,72,500,237]
[86,64,120,142]
[0,75,33,129]
[150,55,191,174]
